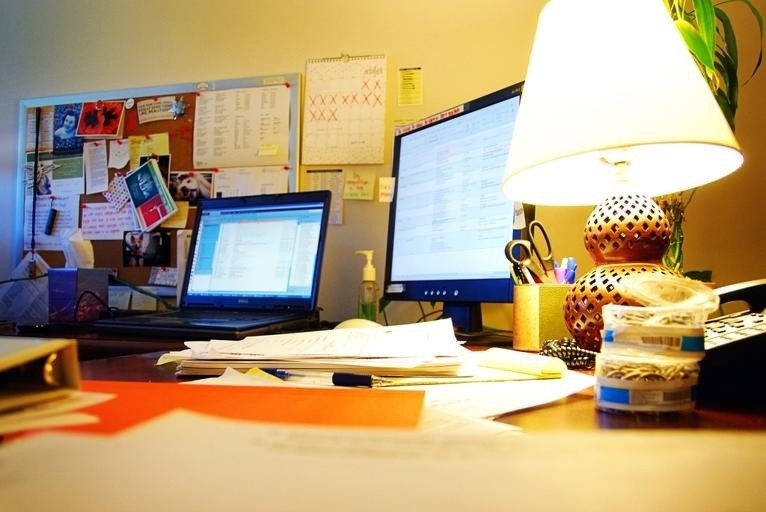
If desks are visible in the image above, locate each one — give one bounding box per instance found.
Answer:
[0,336,766,511]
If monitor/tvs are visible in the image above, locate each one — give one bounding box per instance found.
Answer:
[381,80,537,347]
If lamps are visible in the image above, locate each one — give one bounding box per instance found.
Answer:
[508,0,748,358]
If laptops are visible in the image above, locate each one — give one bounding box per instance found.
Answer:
[94,189,332,338]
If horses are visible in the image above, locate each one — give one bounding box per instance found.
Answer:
[176,171,211,200]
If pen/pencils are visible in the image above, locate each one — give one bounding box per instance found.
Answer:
[508,257,577,284]
[260,368,394,387]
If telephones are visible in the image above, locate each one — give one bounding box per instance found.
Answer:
[691,278,766,406]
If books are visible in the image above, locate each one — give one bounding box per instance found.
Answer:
[152,314,481,386]
[0,334,83,410]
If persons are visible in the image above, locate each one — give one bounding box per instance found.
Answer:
[54,109,83,142]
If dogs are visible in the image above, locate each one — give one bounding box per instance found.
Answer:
[128,233,144,266]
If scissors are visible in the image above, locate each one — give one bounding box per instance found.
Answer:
[505,220,558,284]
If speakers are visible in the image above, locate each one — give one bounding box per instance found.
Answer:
[48,268,110,322]
